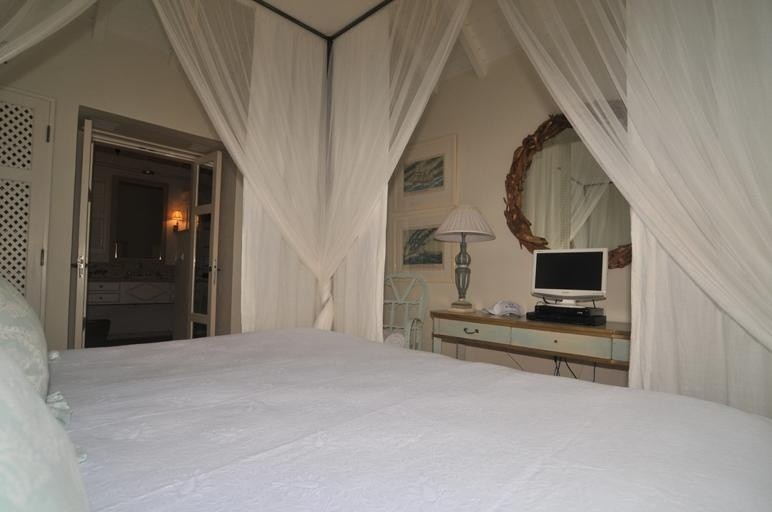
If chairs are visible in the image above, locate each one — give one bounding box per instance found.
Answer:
[383,274,429,351]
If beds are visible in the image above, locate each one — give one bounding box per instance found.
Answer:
[51,331,770,512]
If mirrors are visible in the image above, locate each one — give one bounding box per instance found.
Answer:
[501,98,629,267]
[112,179,170,262]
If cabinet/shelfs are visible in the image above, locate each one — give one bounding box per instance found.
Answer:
[87,280,121,305]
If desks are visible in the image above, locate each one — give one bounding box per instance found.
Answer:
[432,313,630,385]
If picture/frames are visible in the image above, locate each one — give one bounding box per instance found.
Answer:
[392,203,459,285]
[392,131,459,216]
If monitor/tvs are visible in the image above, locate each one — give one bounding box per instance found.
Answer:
[531,247,609,308]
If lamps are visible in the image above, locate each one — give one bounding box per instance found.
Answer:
[435,202,497,313]
[170,209,185,232]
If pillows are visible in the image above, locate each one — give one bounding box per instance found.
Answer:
[0,276,54,399]
[0,347,90,511]
